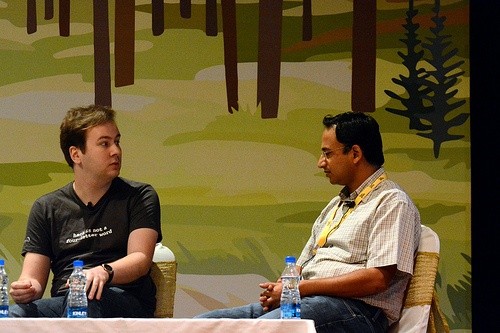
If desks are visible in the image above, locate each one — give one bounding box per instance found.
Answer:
[0,317,316,333]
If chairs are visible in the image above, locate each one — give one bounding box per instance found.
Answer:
[390,225,440,333]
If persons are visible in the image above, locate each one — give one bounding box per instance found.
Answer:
[8,104,163,318]
[191,109,422,333]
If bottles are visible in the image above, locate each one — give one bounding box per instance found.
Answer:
[67,260,88,318]
[280,256,301,319]
[0,260,9,319]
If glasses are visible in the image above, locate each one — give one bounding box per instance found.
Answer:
[320,146,345,161]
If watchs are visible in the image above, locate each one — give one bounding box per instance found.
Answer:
[100,261,114,285]
